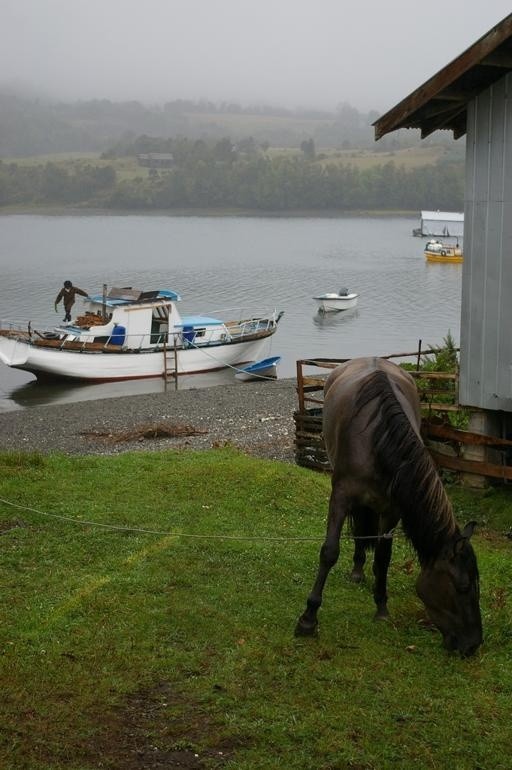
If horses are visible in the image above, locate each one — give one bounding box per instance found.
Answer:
[290,354,485,659]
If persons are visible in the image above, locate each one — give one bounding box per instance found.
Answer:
[54,280,89,322]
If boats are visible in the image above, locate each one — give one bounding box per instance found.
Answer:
[312,308,357,328]
[422,235,463,264]
[1,277,286,378]
[235,353,282,382]
[413,205,465,239]
[311,285,359,312]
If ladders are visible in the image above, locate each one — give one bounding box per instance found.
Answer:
[163,339,178,393]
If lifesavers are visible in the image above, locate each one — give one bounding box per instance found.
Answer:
[440,251,446,257]
[44,332,59,340]
